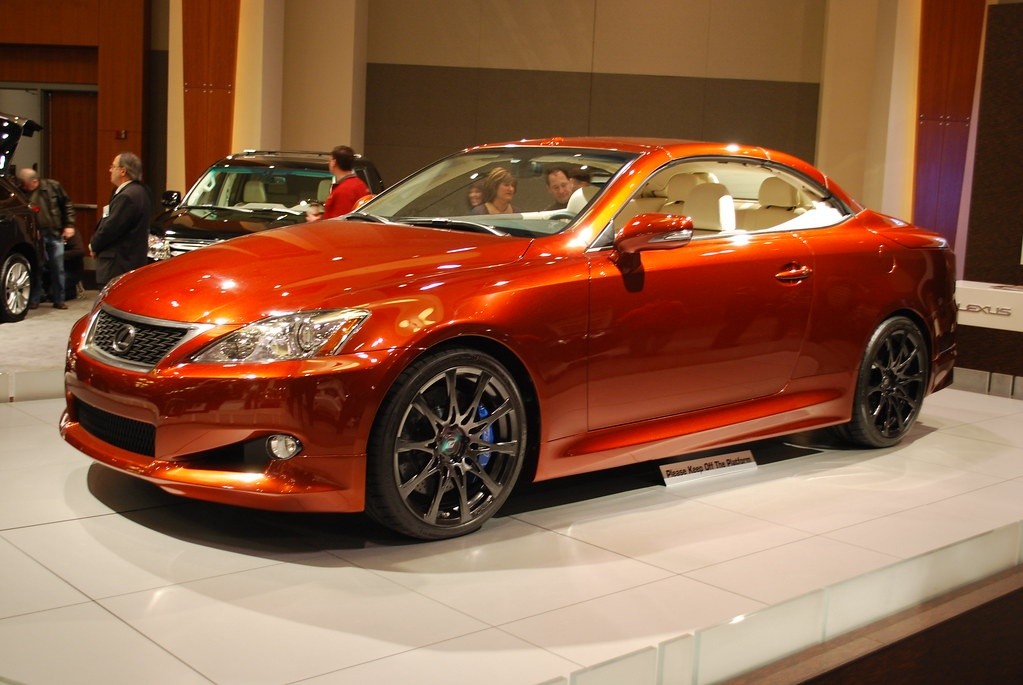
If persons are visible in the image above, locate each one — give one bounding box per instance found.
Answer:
[546,164,573,211]
[468,177,488,207]
[470,166,525,216]
[64,231,85,302]
[17,168,75,310]
[87,153,153,292]
[320,146,370,220]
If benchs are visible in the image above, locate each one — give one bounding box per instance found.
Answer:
[657,173,809,230]
[267,176,299,202]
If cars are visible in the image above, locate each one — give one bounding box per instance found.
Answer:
[0,112,43,321]
[60,137,958,540]
[146,151,386,261]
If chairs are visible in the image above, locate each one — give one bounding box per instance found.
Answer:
[233,180,266,206]
[317,179,332,204]
[564,186,601,222]
[685,183,735,235]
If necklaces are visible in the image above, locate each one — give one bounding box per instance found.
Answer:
[492,203,508,212]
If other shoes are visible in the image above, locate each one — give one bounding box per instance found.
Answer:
[29,300,38,309]
[53,303,67,309]
